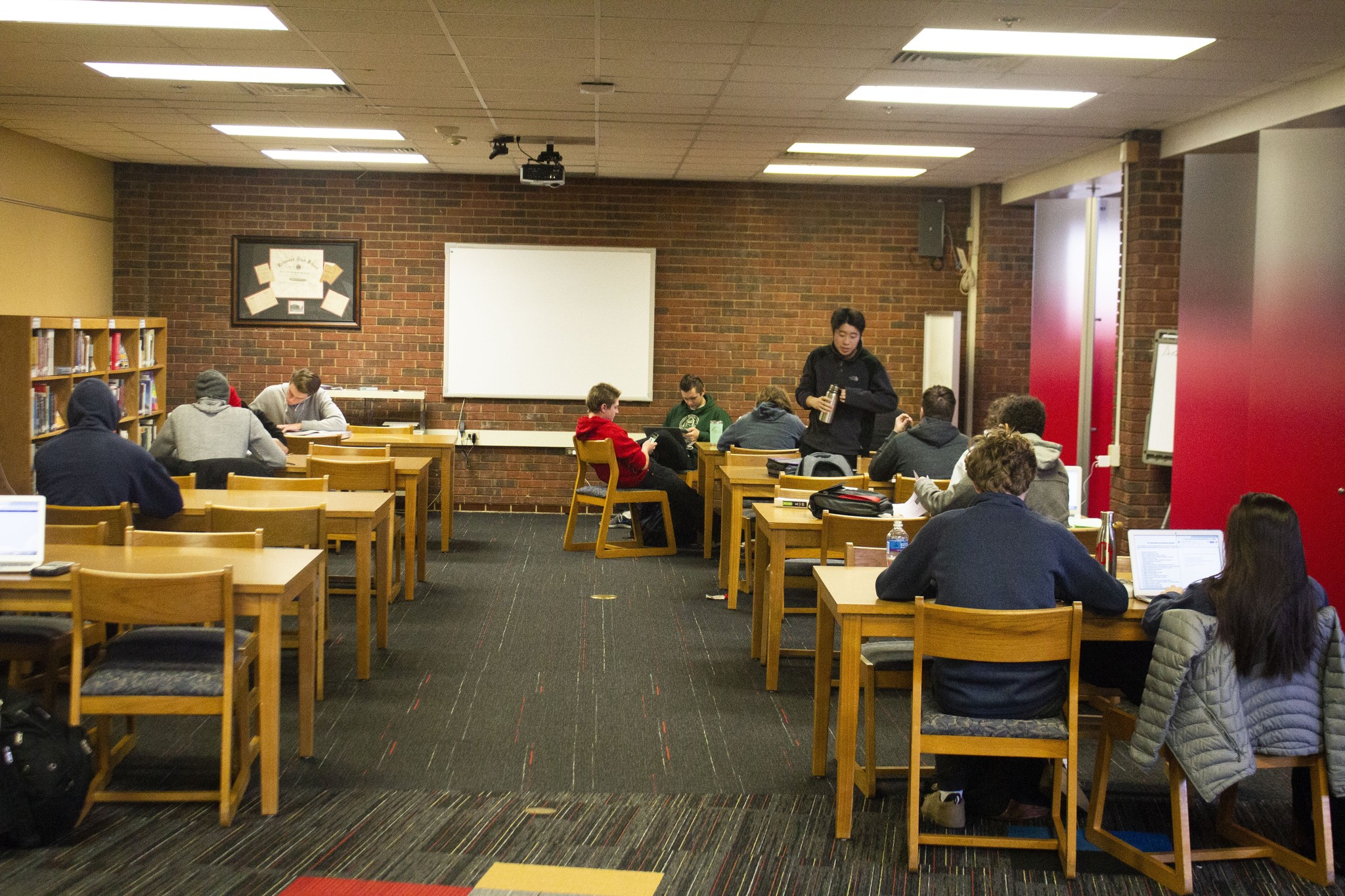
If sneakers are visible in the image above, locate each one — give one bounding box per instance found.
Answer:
[599,512,633,529]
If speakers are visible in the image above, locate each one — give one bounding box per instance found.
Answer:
[919,202,946,256]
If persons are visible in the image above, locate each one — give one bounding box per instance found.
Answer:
[248,369,347,434]
[596,374,732,528]
[34,377,184,528]
[869,385,971,482]
[575,383,722,537]
[1142,491,1330,679]
[795,307,911,469]
[875,428,1129,827]
[717,385,808,456]
[913,395,1069,527]
[147,369,289,470]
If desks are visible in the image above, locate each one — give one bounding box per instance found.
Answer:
[342,431,459,556]
[718,464,897,611]
[805,561,1159,843]
[284,447,433,603]
[749,498,1115,694]
[695,440,879,559]
[1,541,324,816]
[138,486,395,681]
[323,388,426,433]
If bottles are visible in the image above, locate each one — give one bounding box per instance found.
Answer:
[886,520,908,568]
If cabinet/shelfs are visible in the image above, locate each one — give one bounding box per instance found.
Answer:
[0,315,168,494]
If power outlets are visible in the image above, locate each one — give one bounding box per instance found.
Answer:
[1108,445,1122,470]
[463,430,480,441]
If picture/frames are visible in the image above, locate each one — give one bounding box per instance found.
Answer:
[229,233,362,331]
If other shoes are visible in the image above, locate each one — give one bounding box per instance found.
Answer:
[919,791,967,829]
[999,800,1048,820]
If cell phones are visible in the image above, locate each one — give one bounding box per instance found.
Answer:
[680,429,689,433]
[649,432,660,443]
[31,562,75,577]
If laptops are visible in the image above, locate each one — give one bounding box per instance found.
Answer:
[642,426,697,448]
[0,495,47,573]
[1127,529,1226,603]
[1065,465,1084,526]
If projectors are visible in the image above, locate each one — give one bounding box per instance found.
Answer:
[520,164,566,188]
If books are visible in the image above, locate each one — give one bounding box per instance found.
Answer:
[32,329,159,469]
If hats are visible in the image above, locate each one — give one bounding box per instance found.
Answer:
[194,369,229,404]
[229,385,242,408]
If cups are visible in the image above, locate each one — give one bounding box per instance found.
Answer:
[710,420,723,446]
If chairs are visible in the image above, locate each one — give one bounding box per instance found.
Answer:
[0,425,1344,896]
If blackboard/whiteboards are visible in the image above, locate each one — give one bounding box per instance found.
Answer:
[442,242,656,402]
[1140,327,1178,466]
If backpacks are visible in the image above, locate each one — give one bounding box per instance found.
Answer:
[0,702,94,846]
[795,452,855,477]
[766,456,797,478]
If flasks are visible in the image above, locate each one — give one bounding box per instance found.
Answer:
[1096,511,1116,580]
[819,384,841,424]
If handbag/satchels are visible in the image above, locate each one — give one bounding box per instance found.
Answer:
[807,482,894,520]
